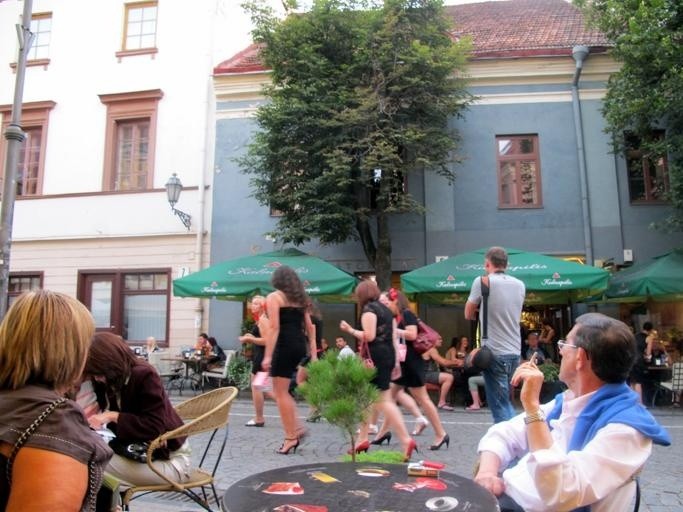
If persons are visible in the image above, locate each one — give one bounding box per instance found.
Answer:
[0,290,114,512]
[472,313,672,512]
[628,322,683,409]
[75,332,192,512]
[145,337,160,353]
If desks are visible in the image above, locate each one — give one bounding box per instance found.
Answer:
[220,459,501,511]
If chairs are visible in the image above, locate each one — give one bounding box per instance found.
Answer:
[422,354,683,410]
[119,344,239,511]
[473,453,642,511]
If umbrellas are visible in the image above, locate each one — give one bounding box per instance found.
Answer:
[400,246,610,310]
[172,245,361,311]
[584,245,683,306]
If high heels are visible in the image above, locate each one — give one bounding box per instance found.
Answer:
[403,439,419,463]
[370,431,391,446]
[428,433,450,451]
[276,409,321,455]
[347,439,370,455]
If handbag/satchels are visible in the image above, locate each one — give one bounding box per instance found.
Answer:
[252,367,273,393]
[357,332,374,368]
[401,309,443,354]
[107,435,170,464]
[396,330,408,362]
[465,343,494,372]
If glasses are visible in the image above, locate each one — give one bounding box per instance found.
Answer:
[556,338,589,361]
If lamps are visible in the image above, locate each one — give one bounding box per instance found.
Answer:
[163,172,191,231]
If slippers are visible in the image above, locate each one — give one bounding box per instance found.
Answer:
[245,419,264,427]
[438,403,484,412]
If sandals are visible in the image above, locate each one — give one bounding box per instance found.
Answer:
[410,414,428,436]
[357,424,378,435]
